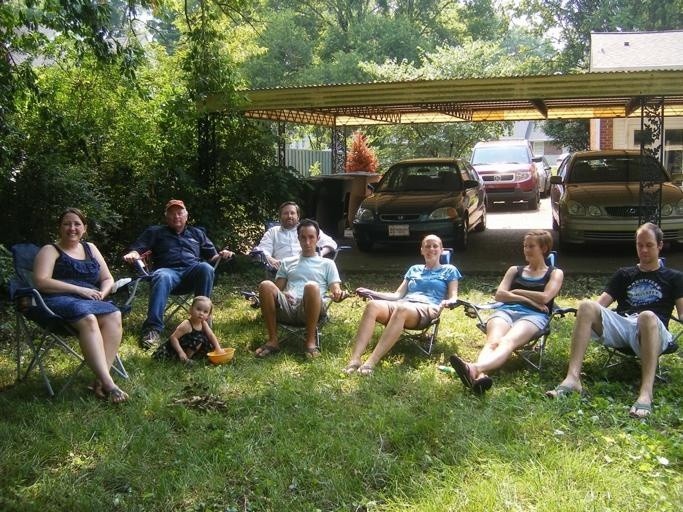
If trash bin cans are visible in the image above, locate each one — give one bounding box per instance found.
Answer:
[299,173,383,241]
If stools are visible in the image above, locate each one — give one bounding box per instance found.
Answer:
[122,240,235,323]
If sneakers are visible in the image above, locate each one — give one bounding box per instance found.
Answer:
[140,329,159,349]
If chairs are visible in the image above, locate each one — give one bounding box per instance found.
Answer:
[450,250,573,370]
[570,259,683,379]
[256,288,333,351]
[361,246,455,358]
[7,237,138,401]
[249,241,346,275]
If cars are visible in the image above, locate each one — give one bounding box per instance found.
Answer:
[547,149,683,251]
[351,158,486,255]
[534,155,550,197]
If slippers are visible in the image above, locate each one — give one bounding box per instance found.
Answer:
[629,400,654,419]
[303,346,322,361]
[449,353,473,388]
[544,384,584,398]
[345,364,376,379]
[472,377,493,396]
[254,343,280,358]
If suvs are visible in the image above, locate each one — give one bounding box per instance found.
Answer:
[468,137,541,211]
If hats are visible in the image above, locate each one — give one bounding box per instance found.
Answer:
[165,199,186,211]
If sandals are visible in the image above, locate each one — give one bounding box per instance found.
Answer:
[86,379,130,404]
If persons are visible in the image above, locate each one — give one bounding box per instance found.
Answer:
[147,295,224,370]
[252,198,338,274]
[31,206,131,405]
[448,228,564,394]
[120,197,237,351]
[544,222,682,420]
[341,233,464,378]
[250,217,350,363]
